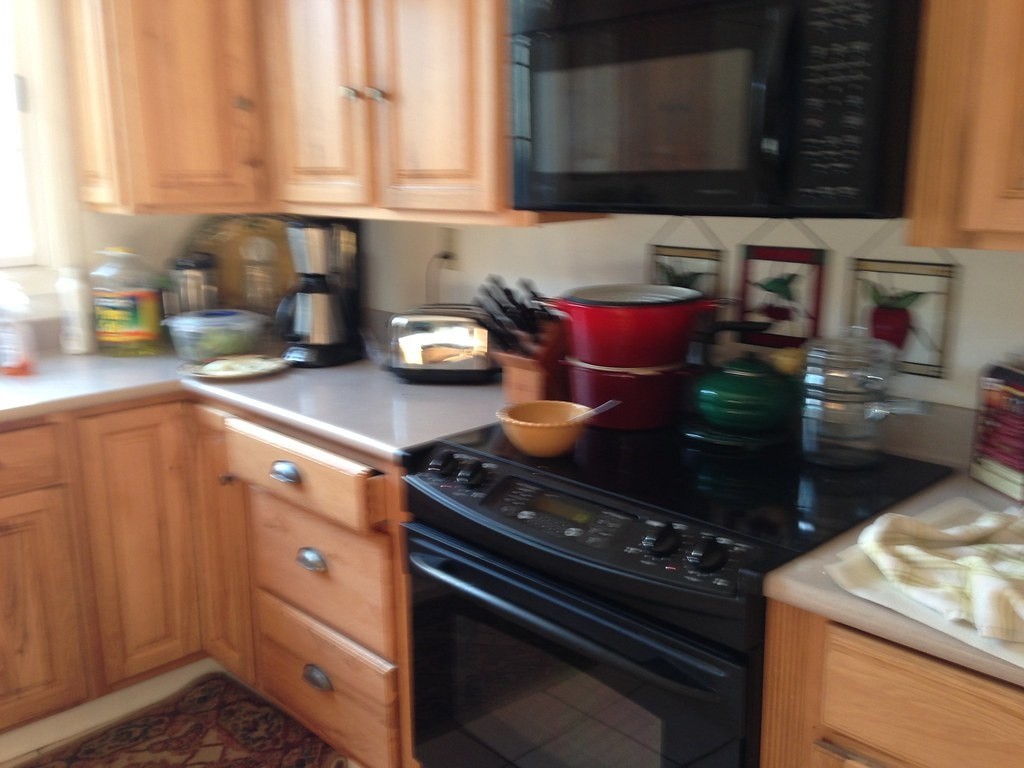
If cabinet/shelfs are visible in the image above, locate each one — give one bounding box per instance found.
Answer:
[758,600,1023,768]
[0,390,203,734]
[188,393,420,768]
[57,0,269,219]
[257,0,614,228]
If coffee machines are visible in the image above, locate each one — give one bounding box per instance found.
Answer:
[276,218,358,365]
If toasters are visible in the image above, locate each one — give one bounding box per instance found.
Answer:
[388,304,491,386]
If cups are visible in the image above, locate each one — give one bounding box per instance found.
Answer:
[167,252,219,314]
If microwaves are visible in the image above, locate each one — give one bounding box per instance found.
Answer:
[512,0,919,218]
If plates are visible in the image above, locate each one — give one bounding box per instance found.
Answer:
[175,353,289,379]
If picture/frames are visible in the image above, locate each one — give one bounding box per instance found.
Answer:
[729,241,828,350]
[642,242,721,299]
[842,255,956,382]
[905,0,1024,253]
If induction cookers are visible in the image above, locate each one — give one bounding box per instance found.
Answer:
[404,403,951,554]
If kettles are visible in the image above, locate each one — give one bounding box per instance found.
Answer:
[691,358,806,430]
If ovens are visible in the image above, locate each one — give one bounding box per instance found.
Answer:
[399,501,757,768]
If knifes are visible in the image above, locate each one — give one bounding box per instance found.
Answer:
[474,276,556,354]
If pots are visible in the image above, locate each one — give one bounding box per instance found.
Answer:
[532,285,736,368]
[557,356,710,432]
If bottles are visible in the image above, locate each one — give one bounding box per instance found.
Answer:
[94,247,161,354]
[804,324,895,465]
[0,275,33,376]
[59,269,94,354]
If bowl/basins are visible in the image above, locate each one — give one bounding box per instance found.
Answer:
[161,307,271,365]
[497,401,594,459]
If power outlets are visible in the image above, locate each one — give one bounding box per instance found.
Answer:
[439,228,462,271]
